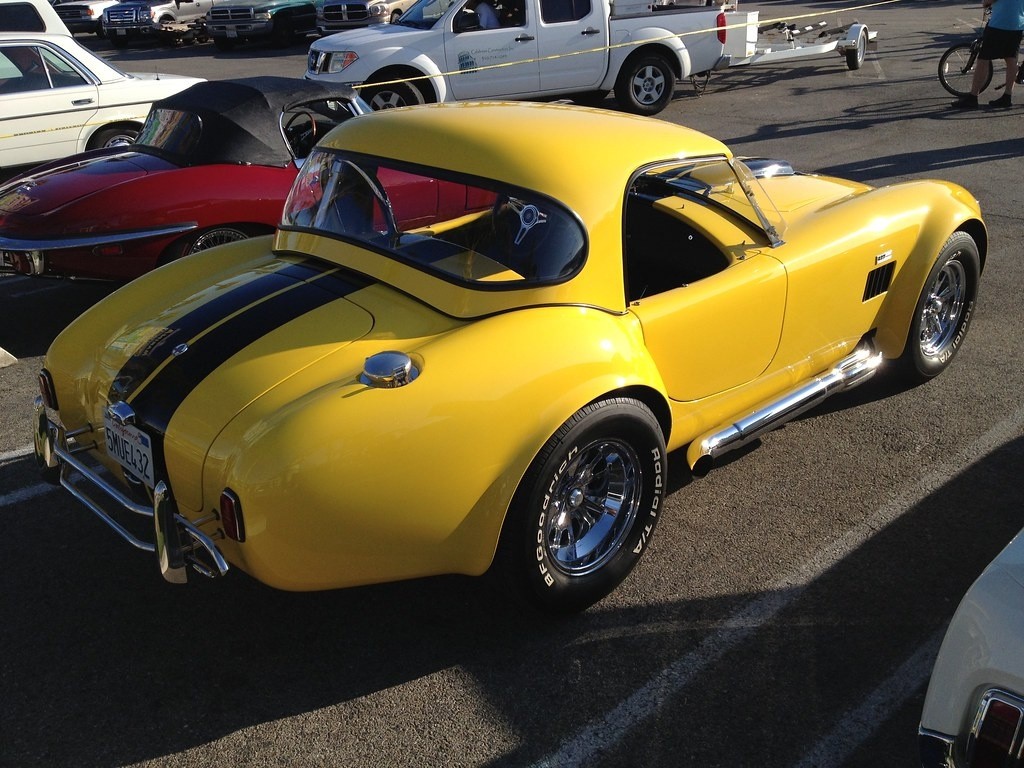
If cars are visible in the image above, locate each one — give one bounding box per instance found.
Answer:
[30,96,991,623]
[1,31,211,170]
[915,529,1024,768]
[0,72,508,361]
[44,0,455,54]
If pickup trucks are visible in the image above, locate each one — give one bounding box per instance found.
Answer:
[304,0,759,114]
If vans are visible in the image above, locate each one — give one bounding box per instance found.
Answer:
[0,0,85,75]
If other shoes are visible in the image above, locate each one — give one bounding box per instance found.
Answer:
[951,93,979,109]
[989,93,1012,106]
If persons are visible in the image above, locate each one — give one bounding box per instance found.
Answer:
[951,0,1024,108]
[464,0,499,29]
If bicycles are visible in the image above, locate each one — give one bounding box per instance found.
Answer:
[937,4,1024,98]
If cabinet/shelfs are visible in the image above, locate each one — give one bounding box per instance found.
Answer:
[722,11,759,57]
